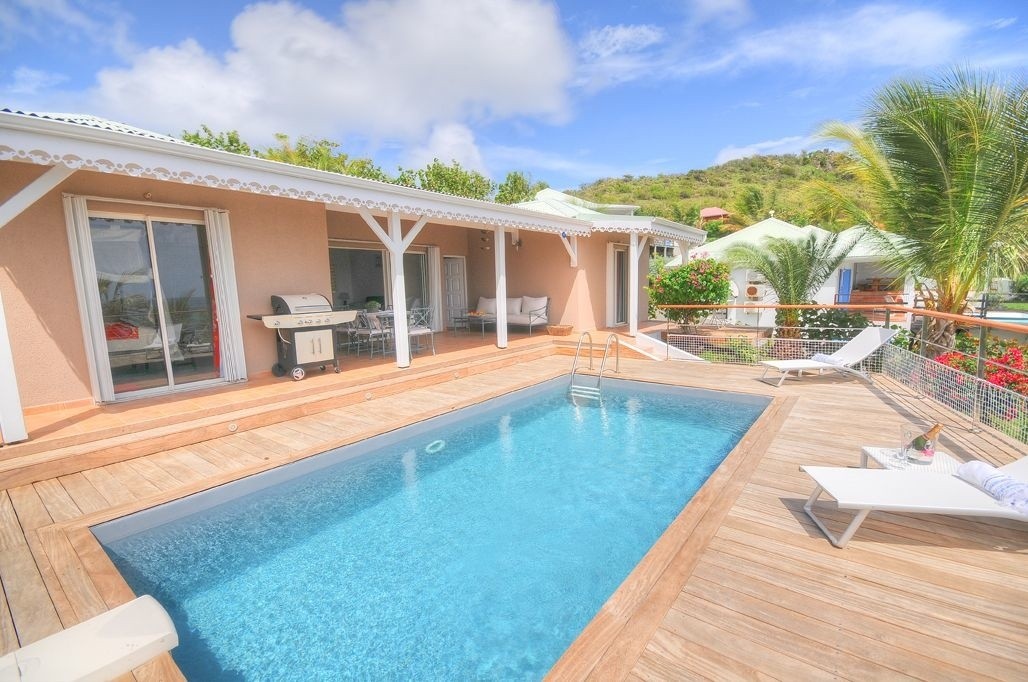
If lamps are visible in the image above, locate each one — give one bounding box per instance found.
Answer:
[339,293,349,304]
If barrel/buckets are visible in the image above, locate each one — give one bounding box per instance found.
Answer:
[900,423,939,465]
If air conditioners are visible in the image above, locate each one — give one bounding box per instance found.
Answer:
[745,269,766,314]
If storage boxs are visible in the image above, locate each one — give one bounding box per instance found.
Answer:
[546,325,572,335]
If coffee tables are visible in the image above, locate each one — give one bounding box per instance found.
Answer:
[453,317,496,337]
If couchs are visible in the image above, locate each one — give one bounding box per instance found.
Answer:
[466,297,549,335]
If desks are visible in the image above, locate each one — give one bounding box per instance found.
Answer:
[371,311,413,352]
[867,278,884,291]
[187,343,211,353]
[861,447,964,469]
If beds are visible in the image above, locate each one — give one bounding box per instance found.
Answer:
[105,322,163,369]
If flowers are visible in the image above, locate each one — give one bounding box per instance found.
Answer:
[643,249,731,333]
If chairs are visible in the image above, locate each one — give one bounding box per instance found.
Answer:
[334,308,436,356]
[146,325,181,357]
[758,326,896,387]
[798,455,1028,548]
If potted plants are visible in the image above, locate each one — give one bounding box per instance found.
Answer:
[365,302,379,312]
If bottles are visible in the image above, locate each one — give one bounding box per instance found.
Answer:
[909,422,943,451]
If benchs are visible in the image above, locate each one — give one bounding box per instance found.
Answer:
[858,282,889,289]
